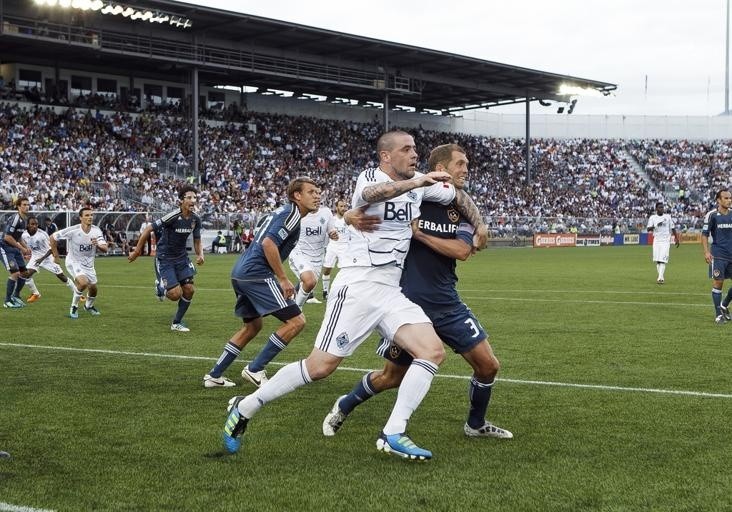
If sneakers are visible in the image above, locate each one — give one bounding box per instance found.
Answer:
[323,394,348,437]
[242,364,268,387]
[3,293,41,308]
[155,280,165,301]
[170,322,190,332]
[464,420,513,439]
[224,396,249,454]
[376,429,432,462]
[204,375,236,387]
[70,295,100,318]
[715,301,731,323]
[306,297,321,303]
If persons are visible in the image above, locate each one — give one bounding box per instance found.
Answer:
[1,76,732,462]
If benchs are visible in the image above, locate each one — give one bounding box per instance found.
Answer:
[95,246,128,258]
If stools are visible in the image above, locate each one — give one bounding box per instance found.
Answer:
[216,245,228,254]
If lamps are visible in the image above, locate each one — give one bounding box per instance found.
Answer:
[29,1,196,30]
